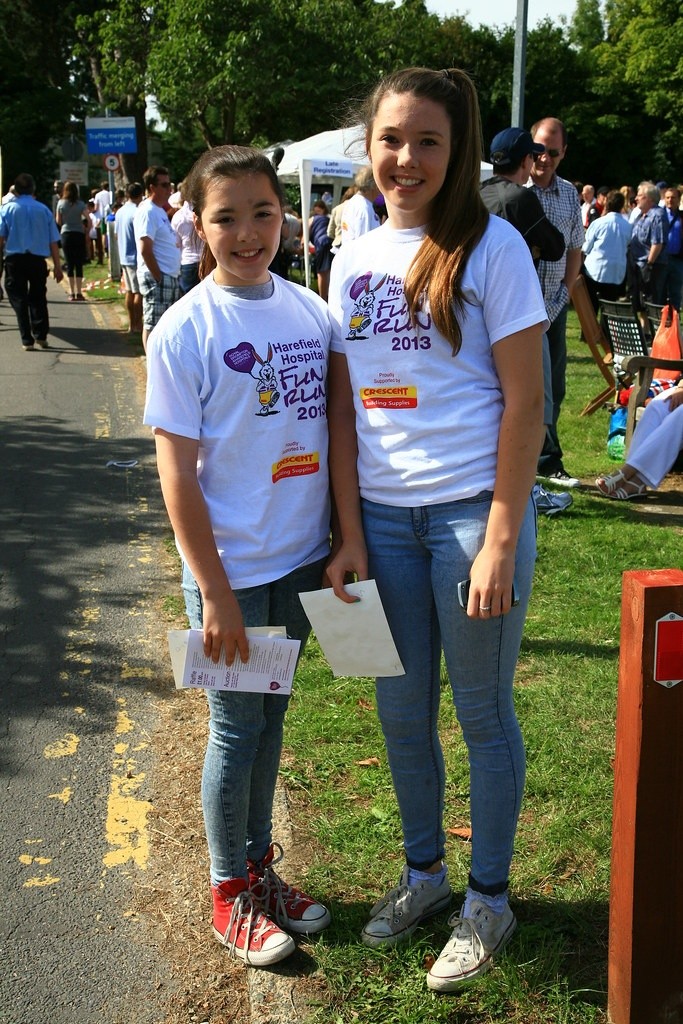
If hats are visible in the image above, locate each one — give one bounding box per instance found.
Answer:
[489,127,545,165]
[597,187,608,194]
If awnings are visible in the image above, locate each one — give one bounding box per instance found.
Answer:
[259,122,534,290]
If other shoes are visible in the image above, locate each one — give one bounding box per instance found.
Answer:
[23,345,34,350]
[36,340,48,347]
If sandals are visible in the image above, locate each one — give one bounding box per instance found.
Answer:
[595,468,648,500]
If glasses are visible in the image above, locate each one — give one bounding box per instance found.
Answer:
[537,146,564,157]
[529,152,538,162]
[157,182,171,188]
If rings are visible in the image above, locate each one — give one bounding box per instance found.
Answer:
[479,606,490,611]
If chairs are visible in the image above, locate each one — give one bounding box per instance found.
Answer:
[621,355,683,450]
[570,274,616,416]
[645,301,663,335]
[598,299,649,357]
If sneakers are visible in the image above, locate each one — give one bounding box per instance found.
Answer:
[536,469,579,487]
[532,482,573,514]
[212,877,295,966]
[425,894,518,991]
[247,841,330,933]
[69,295,84,301]
[361,861,452,949]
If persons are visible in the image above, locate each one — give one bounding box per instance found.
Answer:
[573,181,683,343]
[595,379,683,499]
[479,118,586,514]
[143,144,355,965]
[0,165,388,354]
[326,68,524,991]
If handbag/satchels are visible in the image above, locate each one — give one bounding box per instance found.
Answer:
[651,304,682,380]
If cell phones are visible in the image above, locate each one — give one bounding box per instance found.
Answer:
[458,577,520,610]
